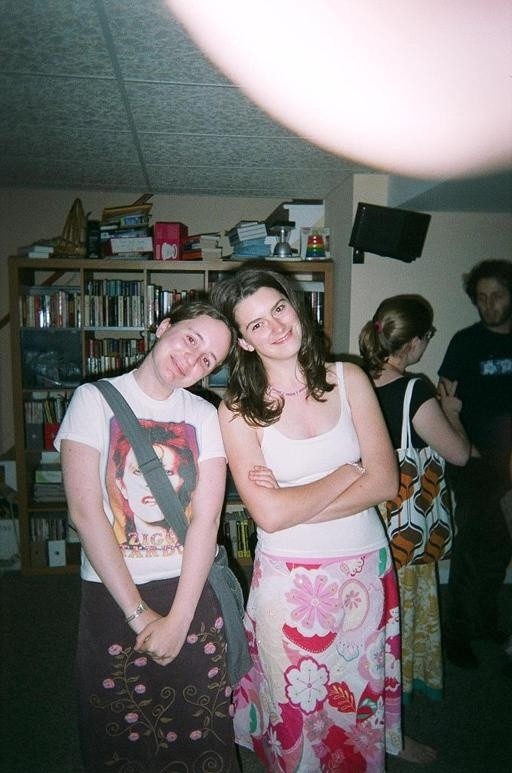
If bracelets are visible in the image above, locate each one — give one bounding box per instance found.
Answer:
[347,462,366,474]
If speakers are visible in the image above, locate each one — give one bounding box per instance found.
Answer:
[348,202,433,263]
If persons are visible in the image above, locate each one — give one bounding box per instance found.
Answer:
[210,261,404,773]
[437,260,511,660]
[358,294,471,765]
[53,300,239,773]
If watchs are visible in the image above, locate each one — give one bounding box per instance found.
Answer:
[124,601,147,623]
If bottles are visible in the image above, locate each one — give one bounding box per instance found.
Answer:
[305,229,327,258]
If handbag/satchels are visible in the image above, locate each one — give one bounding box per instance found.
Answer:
[205,546,253,686]
[378,377,453,570]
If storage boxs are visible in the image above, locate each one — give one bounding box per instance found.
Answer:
[153,221,188,260]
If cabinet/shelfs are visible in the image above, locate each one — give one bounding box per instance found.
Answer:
[9,256,334,576]
